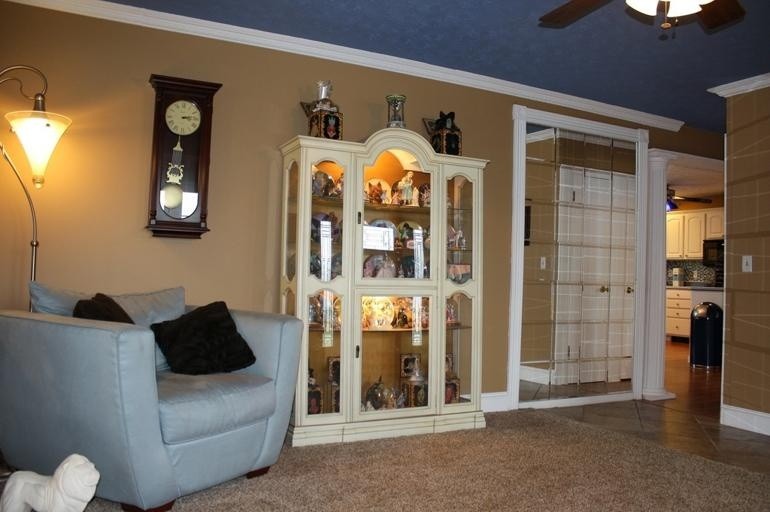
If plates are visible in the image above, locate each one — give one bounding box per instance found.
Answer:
[395,255,428,278]
[363,254,396,278]
[363,296,395,328]
[312,212,340,243]
[393,297,420,329]
[367,218,398,247]
[396,220,423,248]
[364,178,392,208]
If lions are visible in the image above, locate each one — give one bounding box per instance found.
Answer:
[0,450,101,512]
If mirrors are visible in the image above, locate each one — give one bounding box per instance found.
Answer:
[507,104,649,410]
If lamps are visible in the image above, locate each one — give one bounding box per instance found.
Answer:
[625,1,712,17]
[0,65,72,312]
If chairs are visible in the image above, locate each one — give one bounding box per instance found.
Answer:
[1,281,303,511]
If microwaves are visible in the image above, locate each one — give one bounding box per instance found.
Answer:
[702,239,725,266]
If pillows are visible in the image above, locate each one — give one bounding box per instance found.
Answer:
[73,293,257,373]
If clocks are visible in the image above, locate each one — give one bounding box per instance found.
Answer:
[144,74,223,239]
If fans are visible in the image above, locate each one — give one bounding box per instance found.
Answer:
[666,183,713,209]
[537,1,747,35]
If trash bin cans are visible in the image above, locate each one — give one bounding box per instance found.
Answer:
[689,302,723,369]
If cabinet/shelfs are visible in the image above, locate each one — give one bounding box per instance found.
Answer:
[276,128,490,448]
[666,207,724,261]
[666,290,691,338]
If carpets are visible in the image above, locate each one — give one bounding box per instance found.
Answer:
[0,408,770,511]
